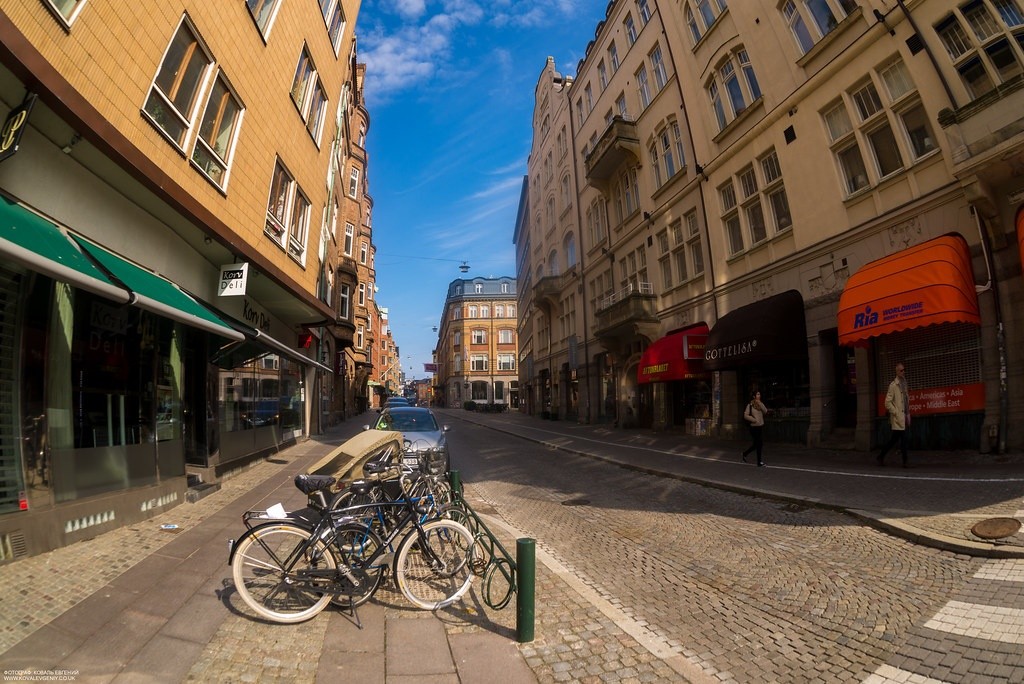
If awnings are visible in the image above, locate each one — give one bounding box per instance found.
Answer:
[0,194,246,341]
[705,290,808,371]
[221,318,334,373]
[1017,209,1024,271]
[837,237,980,349]
[638,326,709,384]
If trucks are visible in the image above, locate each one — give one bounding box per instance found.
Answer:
[403,389,418,406]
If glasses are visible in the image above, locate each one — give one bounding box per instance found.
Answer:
[898,369,907,373]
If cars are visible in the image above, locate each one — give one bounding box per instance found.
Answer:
[364,408,451,477]
[377,396,412,424]
[239,403,284,427]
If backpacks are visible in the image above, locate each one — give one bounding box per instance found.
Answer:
[742,400,751,420]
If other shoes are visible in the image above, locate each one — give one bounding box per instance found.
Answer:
[758,462,767,467]
[740,451,747,463]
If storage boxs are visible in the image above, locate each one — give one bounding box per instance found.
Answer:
[685,418,711,435]
[694,405,709,418]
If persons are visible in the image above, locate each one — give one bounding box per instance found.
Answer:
[876,362,917,468]
[743,390,768,466]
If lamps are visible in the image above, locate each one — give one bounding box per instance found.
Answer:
[572,272,580,281]
[696,164,708,182]
[873,9,895,36]
[644,212,654,225]
[602,248,614,262]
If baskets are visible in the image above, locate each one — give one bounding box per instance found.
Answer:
[416,447,447,477]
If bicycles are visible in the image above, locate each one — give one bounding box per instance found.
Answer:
[228,432,481,625]
[19,415,49,486]
